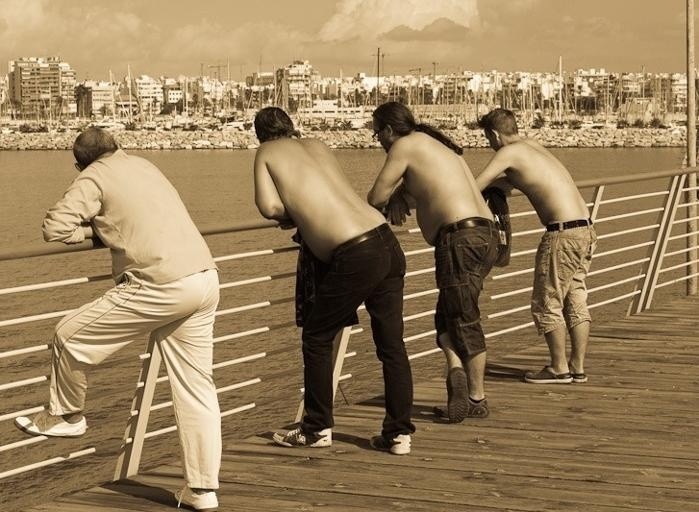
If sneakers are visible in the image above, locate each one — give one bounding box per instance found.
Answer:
[174,485,218,512]
[14,413,88,439]
[371,431,413,455]
[273,427,333,449]
[435,399,489,420]
[445,366,469,423]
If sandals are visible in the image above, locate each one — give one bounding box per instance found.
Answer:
[525,366,588,383]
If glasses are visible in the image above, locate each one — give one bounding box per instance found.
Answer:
[372,127,384,139]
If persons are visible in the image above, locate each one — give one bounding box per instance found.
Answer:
[366,100,501,425]
[473,108,599,384]
[13,125,223,511]
[251,106,417,456]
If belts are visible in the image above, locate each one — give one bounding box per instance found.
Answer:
[547,217,592,233]
[439,218,499,234]
[334,224,389,255]
